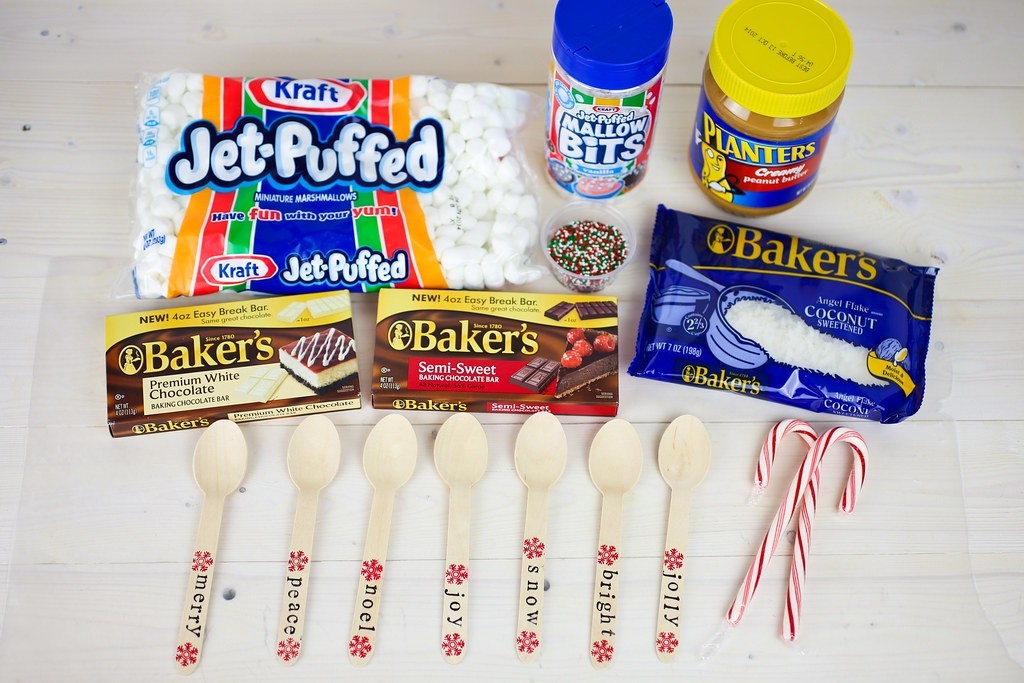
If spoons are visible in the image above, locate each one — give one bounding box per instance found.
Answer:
[654,413,712,665]
[513,409,570,665]
[274,413,342,666]
[587,418,646,671]
[348,414,418,668]
[432,412,490,666]
[173,419,249,676]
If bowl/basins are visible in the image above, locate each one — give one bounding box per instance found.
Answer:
[539,201,639,294]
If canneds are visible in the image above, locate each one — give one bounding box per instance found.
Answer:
[545,0,674,200]
[688,0,854,218]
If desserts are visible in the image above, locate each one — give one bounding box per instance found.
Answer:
[277,328,358,396]
[548,220,627,292]
[508,301,618,398]
